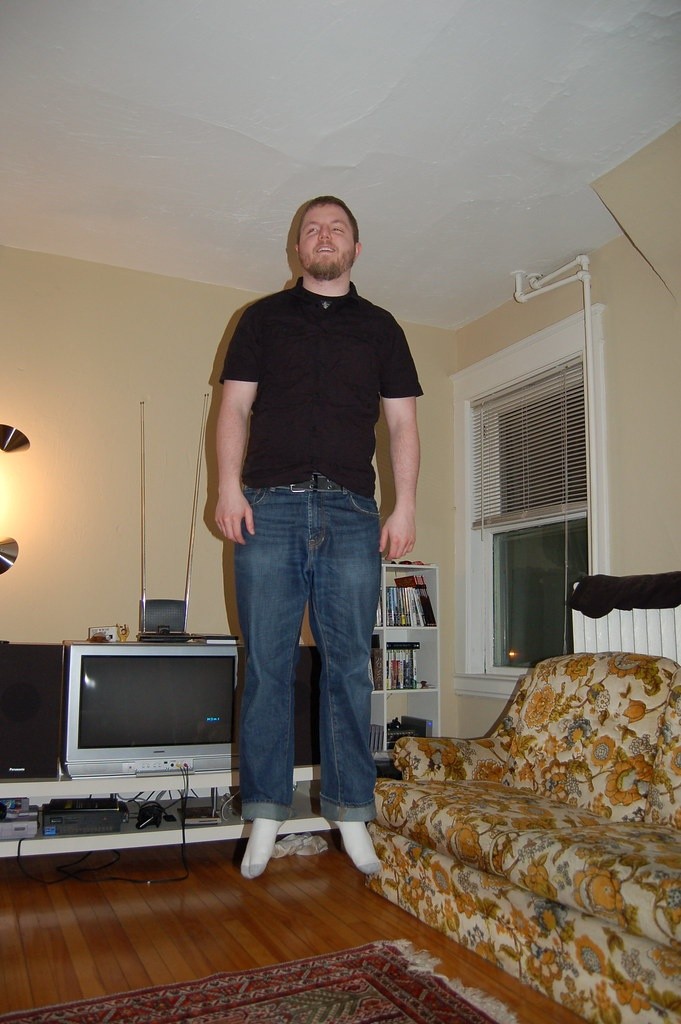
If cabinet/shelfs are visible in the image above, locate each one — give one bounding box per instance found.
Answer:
[371,564,440,761]
[0,757,340,858]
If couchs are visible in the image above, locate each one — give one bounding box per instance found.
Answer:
[365,651,681,1024]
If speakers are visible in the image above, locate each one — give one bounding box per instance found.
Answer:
[0,642,64,779]
[293,646,324,766]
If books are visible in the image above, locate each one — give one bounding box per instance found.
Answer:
[0,798,38,818]
[370,574,437,751]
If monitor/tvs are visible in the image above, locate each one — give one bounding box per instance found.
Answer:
[60,640,244,780]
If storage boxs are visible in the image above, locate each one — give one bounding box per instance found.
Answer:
[90,627,119,643]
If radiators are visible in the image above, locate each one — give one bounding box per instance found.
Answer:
[571,571,681,666]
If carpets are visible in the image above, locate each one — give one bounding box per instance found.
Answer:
[0,938,520,1024]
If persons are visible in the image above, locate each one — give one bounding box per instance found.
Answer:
[118,623,130,643]
[215,196,423,879]
